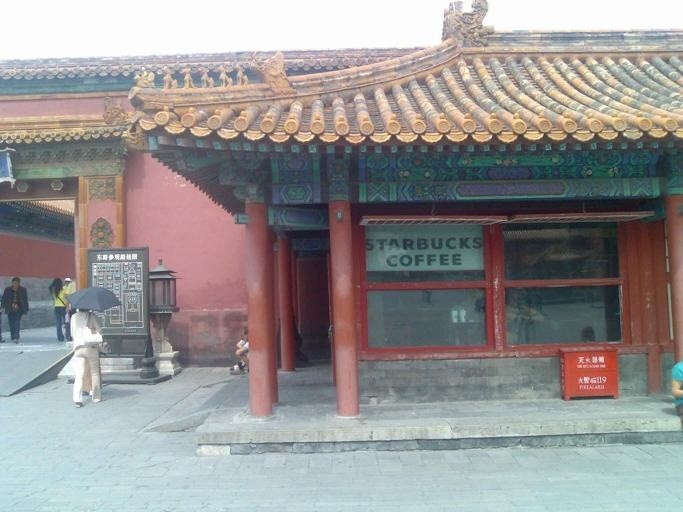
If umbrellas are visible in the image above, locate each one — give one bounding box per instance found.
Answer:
[68,287,122,313]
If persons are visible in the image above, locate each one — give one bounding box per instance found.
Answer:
[579,325,595,340]
[231,326,249,374]
[514,286,545,325]
[48,277,75,343]
[671,357,683,425]
[69,309,105,407]
[424,289,433,305]
[1,275,29,344]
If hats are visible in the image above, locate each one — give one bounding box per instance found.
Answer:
[64,278,72,281]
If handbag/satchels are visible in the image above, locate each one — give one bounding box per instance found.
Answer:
[65,311,72,323]
[82,327,103,344]
